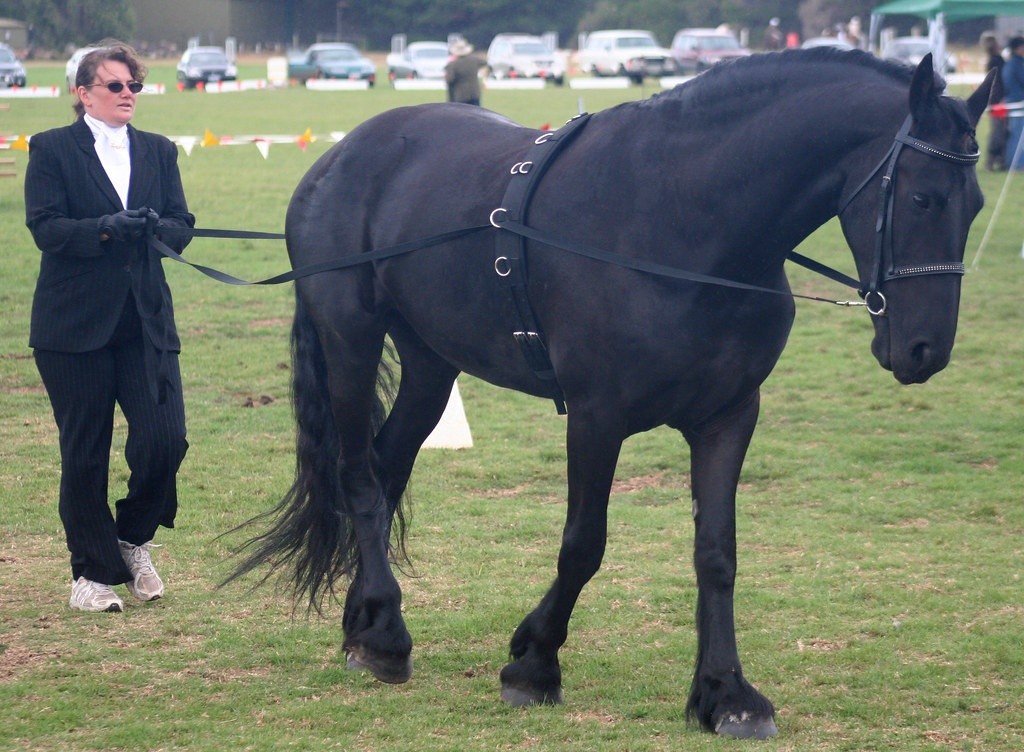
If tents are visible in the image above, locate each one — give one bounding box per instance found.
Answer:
[870,0,1024,83]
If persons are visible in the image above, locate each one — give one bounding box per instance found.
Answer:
[978,32,1024,173]
[445,32,487,108]
[717,16,959,74]
[25,45,195,615]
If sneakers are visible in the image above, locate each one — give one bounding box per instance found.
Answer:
[117,537,164,601]
[69,576,123,612]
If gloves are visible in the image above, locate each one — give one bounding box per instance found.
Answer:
[99,205,159,256]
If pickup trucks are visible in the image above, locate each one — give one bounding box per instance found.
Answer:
[288,43,377,87]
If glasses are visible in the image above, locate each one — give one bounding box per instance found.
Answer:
[84,82,143,93]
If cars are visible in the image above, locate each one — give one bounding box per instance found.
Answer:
[882,35,956,76]
[177,47,236,87]
[798,37,854,54]
[386,41,447,81]
[0,43,28,89]
[65,47,101,89]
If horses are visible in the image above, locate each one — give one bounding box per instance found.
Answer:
[203,50,1003,740]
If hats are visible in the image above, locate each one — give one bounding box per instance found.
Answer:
[449,38,474,57]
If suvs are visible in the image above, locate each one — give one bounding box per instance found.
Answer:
[669,28,750,72]
[586,29,676,78]
[488,31,563,84]
